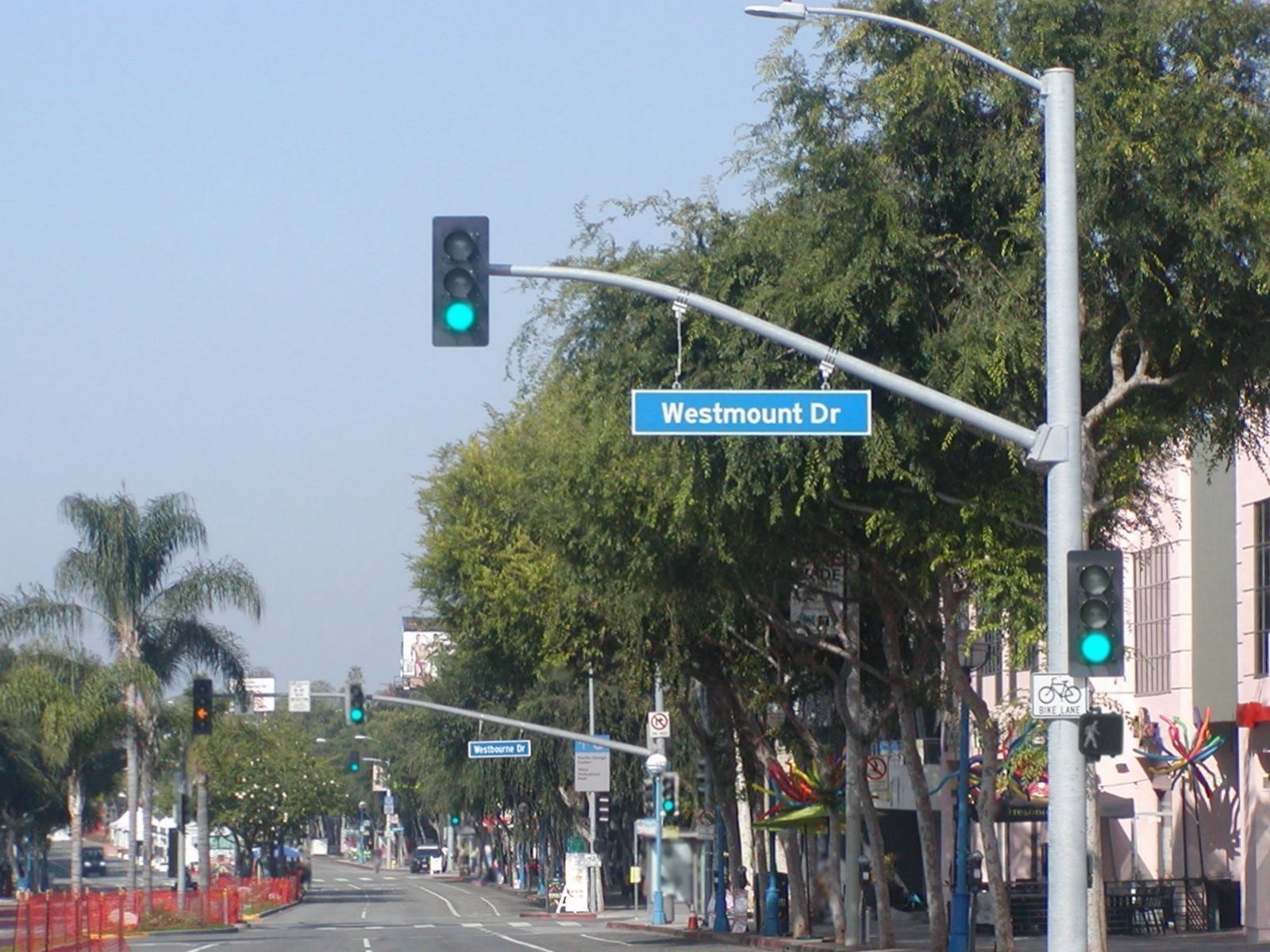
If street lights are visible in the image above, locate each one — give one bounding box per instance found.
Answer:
[744,1,1089,952]
[358,801,368,864]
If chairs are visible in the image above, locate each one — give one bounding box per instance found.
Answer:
[1129,886,1178,936]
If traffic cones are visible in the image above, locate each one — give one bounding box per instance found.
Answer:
[683,903,703,931]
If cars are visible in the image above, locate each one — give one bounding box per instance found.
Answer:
[410,842,449,874]
[81,845,107,876]
[236,840,311,887]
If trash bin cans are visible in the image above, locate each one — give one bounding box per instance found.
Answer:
[753,872,789,934]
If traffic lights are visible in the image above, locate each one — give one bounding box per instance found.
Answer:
[1078,712,1124,757]
[449,815,461,827]
[349,750,360,774]
[659,771,680,816]
[695,757,714,812]
[431,213,489,347]
[346,681,368,725]
[595,796,610,823]
[192,679,213,735]
[1065,549,1125,678]
